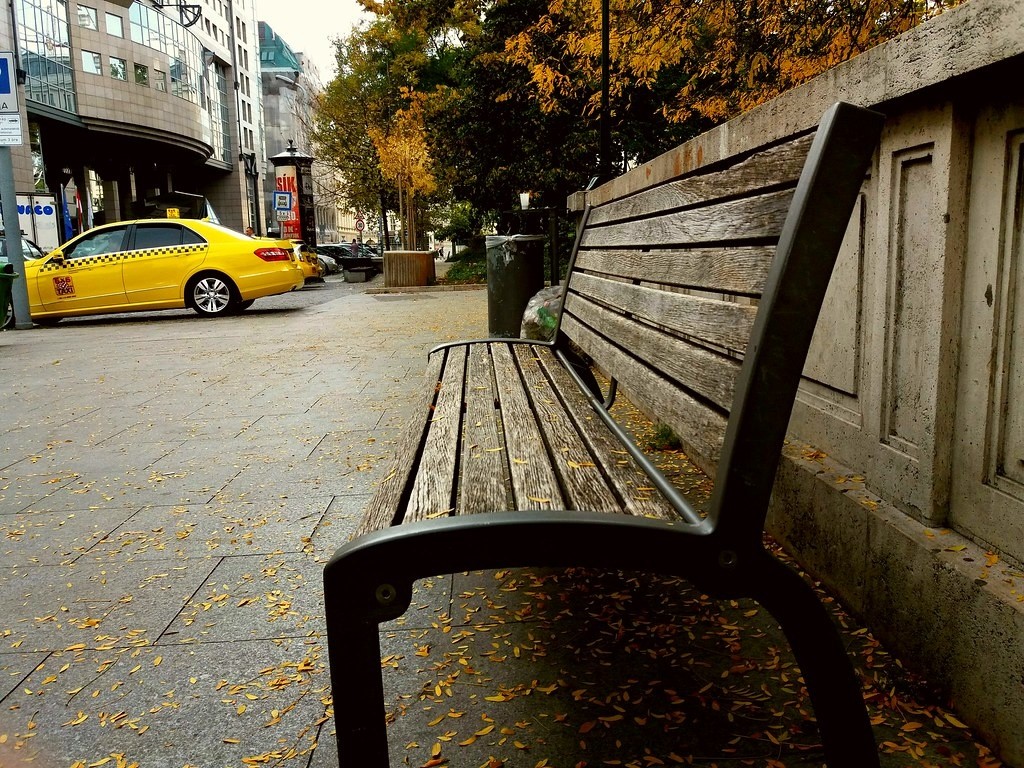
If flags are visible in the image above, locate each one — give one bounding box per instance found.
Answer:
[86,188,94,229]
[62,190,73,240]
[75,190,83,234]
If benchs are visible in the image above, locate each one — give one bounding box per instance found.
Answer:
[324,100,885,768]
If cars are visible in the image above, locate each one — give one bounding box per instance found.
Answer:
[254,238,380,284]
[0,208,304,324]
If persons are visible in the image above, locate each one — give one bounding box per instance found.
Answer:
[350,239,358,257]
[246,227,255,238]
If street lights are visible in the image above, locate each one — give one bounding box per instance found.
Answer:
[274,70,308,153]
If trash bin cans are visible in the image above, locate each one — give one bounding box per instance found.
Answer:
[486,235,544,338]
[0,262,19,331]
